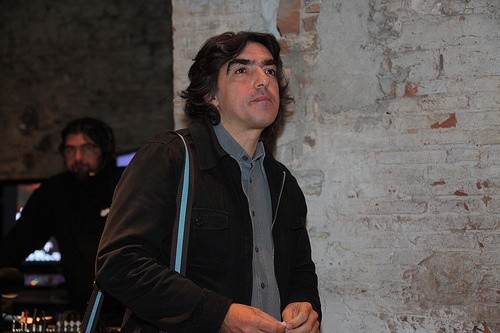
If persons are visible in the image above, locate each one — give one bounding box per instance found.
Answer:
[0,115,126,312]
[93,30,325,333]
